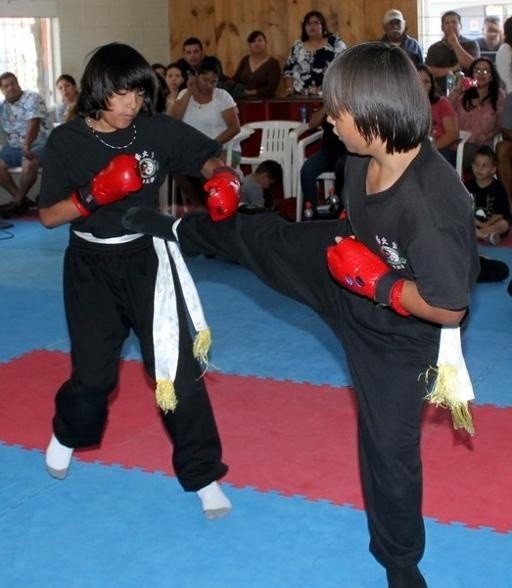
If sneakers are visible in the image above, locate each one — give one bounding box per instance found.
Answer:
[483,232,501,246]
[20,196,36,211]
[0,201,22,218]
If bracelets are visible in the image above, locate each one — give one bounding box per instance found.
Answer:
[285,86,294,91]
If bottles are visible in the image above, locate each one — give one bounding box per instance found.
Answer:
[310,80,318,95]
[328,188,344,218]
[455,68,464,83]
[304,201,314,221]
[462,77,480,88]
[446,70,455,97]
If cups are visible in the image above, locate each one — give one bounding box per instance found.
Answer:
[299,105,307,123]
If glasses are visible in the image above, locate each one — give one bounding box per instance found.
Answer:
[473,68,491,75]
[304,20,321,26]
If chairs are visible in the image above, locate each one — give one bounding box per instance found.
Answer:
[457,131,471,179]
[171,126,250,215]
[298,130,335,222]
[7,167,43,173]
[226,123,308,198]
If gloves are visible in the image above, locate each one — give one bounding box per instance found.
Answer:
[70,154,144,218]
[203,166,242,223]
[326,236,411,318]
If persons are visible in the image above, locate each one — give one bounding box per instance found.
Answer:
[120,41,480,588]
[0,71,54,219]
[56,74,82,123]
[375,7,512,299]
[144,11,348,226]
[36,44,246,521]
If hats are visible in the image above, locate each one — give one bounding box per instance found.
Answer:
[383,9,404,26]
